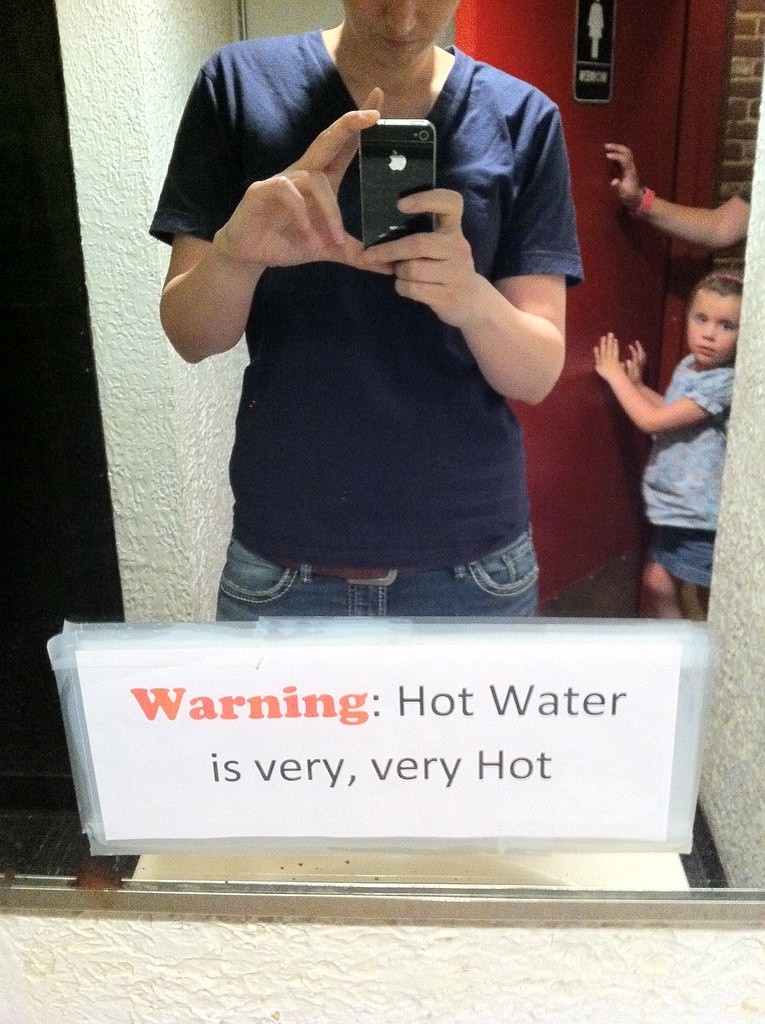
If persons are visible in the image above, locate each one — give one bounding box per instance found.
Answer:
[592,270,742,623]
[148,0,586,621]
[603,141,752,250]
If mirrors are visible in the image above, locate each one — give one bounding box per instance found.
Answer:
[0,0,765,928]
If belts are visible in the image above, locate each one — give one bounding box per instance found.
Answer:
[285,561,446,586]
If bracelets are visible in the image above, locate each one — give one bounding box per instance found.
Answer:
[627,186,655,219]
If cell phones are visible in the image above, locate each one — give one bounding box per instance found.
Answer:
[358,119,437,250]
